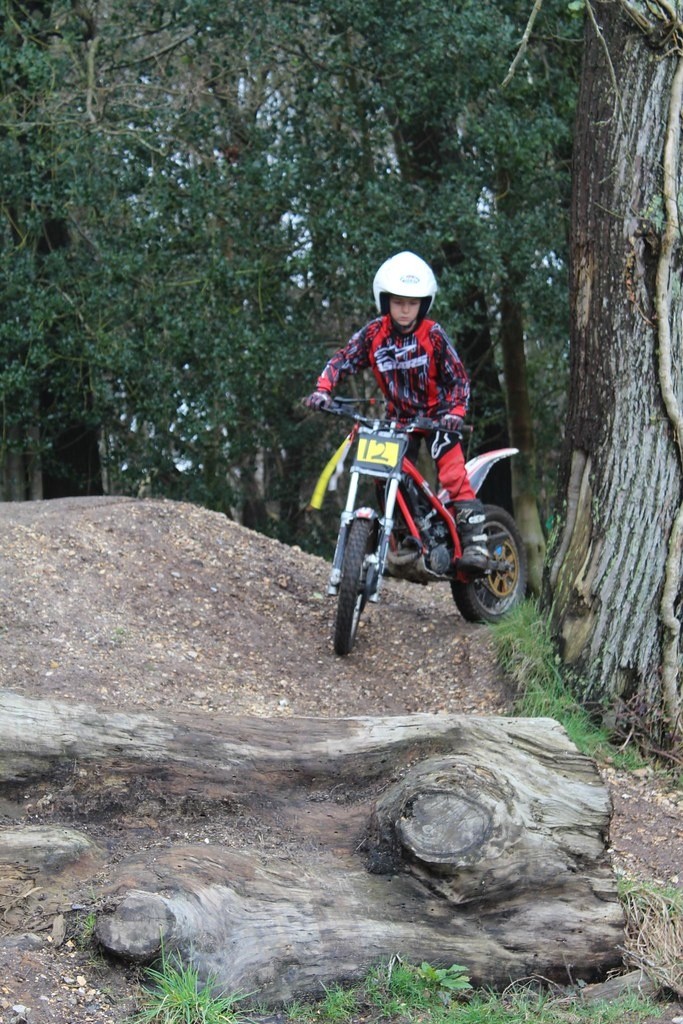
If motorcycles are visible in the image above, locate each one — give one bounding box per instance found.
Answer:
[300,395,528,656]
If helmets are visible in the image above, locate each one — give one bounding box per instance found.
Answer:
[372,249,439,322]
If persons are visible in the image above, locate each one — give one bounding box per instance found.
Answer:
[301,251,490,575]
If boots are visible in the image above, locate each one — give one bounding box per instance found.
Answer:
[456,498,493,572]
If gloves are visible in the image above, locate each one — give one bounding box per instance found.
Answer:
[301,391,333,412]
[442,413,465,432]
